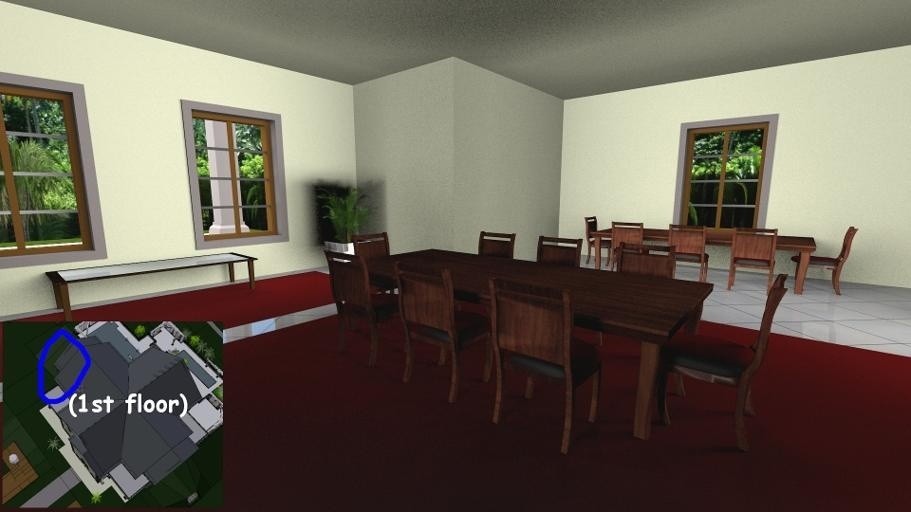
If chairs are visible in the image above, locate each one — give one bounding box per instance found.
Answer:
[488,277,602,455]
[668,224,709,283]
[791,226,858,296]
[617,242,676,279]
[610,221,644,272]
[726,227,778,296]
[536,235,583,267]
[351,232,394,294]
[584,216,610,267]
[321,250,399,368]
[393,261,494,405]
[478,230,516,259]
[657,273,789,452]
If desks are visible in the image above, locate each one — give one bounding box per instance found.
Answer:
[590,228,817,296]
[366,248,714,441]
[44,252,258,320]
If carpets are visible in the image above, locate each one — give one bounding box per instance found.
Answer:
[223,314,911,512]
[0,269,336,383]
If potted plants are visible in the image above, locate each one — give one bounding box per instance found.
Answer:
[317,187,373,263]
